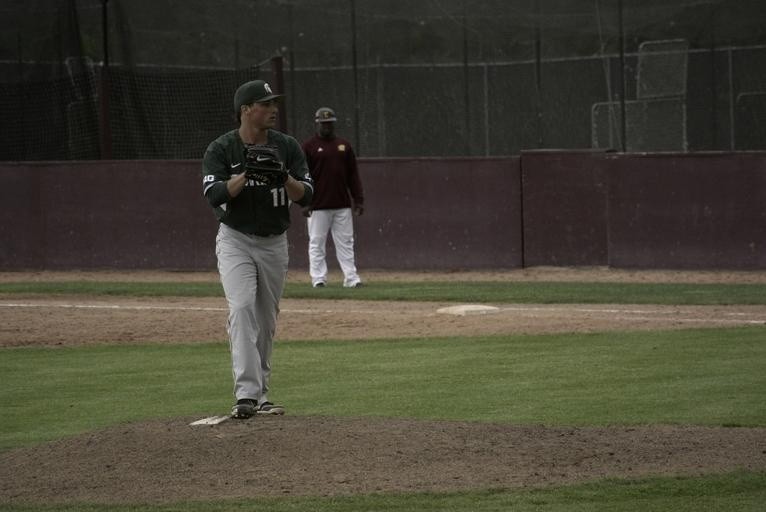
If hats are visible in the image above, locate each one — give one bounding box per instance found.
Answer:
[234,79,281,114]
[315,107,338,123]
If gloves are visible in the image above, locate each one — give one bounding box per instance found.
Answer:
[303,206,312,216]
[353,204,364,215]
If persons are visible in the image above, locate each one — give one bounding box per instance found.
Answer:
[289,107,369,287]
[200,79,313,418]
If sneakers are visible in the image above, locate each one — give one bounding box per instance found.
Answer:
[311,273,364,288]
[232,400,285,419]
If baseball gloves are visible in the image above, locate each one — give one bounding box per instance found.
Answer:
[241,140,287,188]
